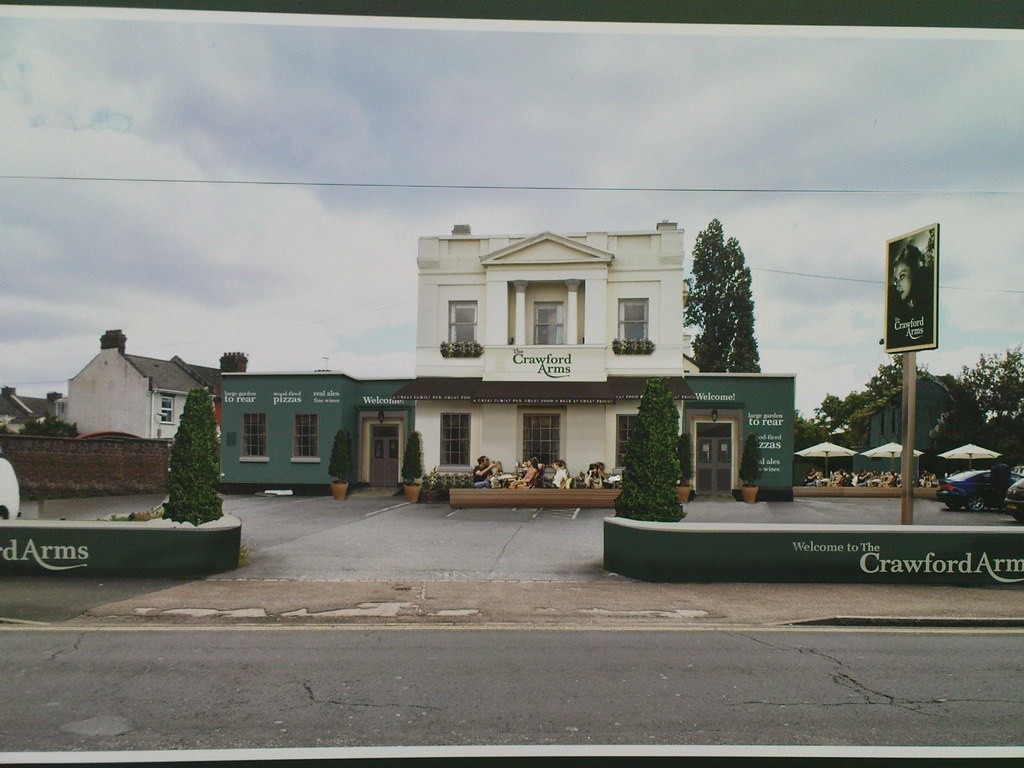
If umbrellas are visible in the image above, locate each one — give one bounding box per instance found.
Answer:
[937,444,1002,470]
[859,442,925,471]
[794,442,858,479]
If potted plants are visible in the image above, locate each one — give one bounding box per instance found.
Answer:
[328,429,351,501]
[675,433,691,505]
[400,431,423,503]
[739,435,763,505]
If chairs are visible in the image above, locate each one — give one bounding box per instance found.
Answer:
[533,464,545,488]
[890,476,897,487]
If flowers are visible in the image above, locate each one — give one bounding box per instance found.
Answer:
[610,339,655,350]
[440,342,484,352]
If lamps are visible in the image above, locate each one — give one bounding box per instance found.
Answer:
[378,411,384,424]
[711,409,718,422]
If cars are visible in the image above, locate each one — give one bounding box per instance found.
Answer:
[934,469,1024,512]
[1004,477,1024,523]
[0,457,21,521]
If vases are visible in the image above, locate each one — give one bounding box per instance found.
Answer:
[614,349,653,354]
[441,351,481,357]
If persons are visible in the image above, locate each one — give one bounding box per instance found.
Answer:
[893,245,932,312]
[510,458,540,489]
[991,456,1011,513]
[805,468,937,488]
[549,460,609,490]
[473,456,495,489]
